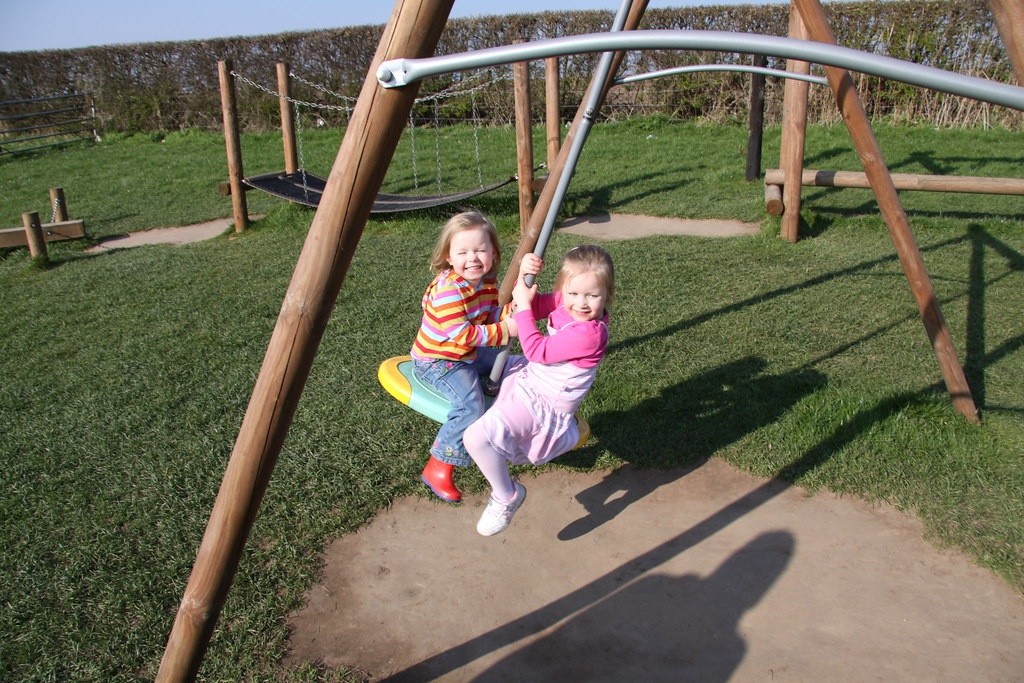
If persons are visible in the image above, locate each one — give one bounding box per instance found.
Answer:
[463,245,614,538]
[410,212,518,503]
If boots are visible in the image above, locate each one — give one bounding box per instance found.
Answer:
[420,455,462,502]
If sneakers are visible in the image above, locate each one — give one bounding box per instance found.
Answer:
[477,476,527,536]
